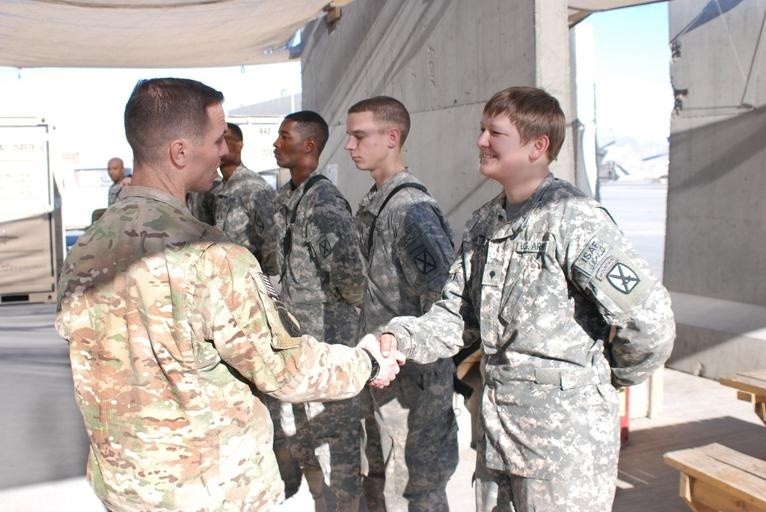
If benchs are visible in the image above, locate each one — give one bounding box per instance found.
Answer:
[661,441,766,512]
[719,370,766,427]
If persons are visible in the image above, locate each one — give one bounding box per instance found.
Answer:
[368,84,682,511]
[211,119,302,507]
[267,110,371,512]
[106,156,129,210]
[52,75,409,512]
[342,95,466,511]
[181,168,230,231]
[118,174,132,191]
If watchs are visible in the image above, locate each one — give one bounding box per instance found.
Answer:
[361,346,382,384]
